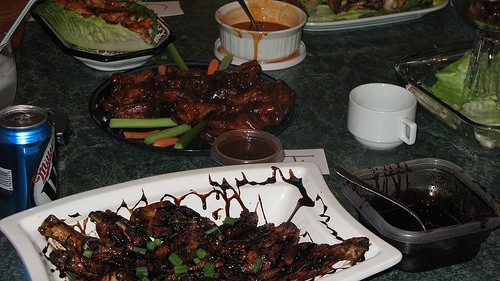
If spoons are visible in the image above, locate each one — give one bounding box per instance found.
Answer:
[334,166,458,249]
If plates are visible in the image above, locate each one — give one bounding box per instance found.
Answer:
[89,61,298,152]
[24,0,175,71]
[0,161,403,281]
[392,38,500,156]
[302,0,451,32]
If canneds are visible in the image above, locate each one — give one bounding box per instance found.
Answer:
[0,105,58,220]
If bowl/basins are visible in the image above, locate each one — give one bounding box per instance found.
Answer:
[450,0,500,34]
[215,0,309,62]
[341,158,500,273]
[211,129,286,166]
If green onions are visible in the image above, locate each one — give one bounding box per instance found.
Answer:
[83,216,263,281]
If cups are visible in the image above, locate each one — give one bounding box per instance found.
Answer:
[0,31,18,113]
[347,83,418,151]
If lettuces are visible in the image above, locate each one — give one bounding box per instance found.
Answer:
[426,49,500,149]
[305,0,444,21]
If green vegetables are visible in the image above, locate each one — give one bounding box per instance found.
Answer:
[33,2,160,51]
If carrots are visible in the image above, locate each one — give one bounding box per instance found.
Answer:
[123,130,161,139]
[152,137,179,148]
[207,59,219,75]
